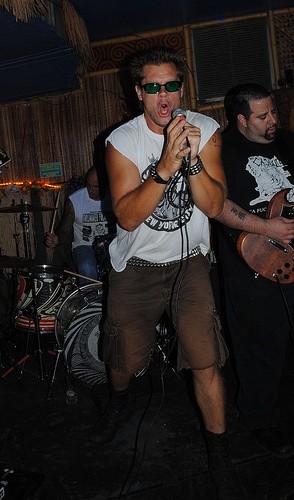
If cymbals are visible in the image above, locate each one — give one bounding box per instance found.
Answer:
[0,205,60,213]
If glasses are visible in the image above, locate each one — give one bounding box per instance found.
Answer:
[138,81,183,94]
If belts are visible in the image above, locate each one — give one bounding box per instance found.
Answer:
[127,246,201,267]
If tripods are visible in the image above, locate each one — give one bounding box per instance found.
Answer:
[10,186,66,385]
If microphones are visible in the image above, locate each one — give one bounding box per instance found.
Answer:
[171,108,191,163]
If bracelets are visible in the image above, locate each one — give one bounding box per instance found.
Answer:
[182,155,203,175]
[150,161,174,184]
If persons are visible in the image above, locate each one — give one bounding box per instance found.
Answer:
[82,45,249,500]
[46,165,117,287]
[209,83,294,458]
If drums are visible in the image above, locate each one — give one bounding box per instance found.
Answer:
[7,264,79,334]
[0,255,38,304]
[53,282,168,393]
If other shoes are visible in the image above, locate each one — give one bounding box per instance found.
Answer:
[102,406,127,440]
[204,430,233,477]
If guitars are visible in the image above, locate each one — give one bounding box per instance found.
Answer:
[236,188,294,284]
